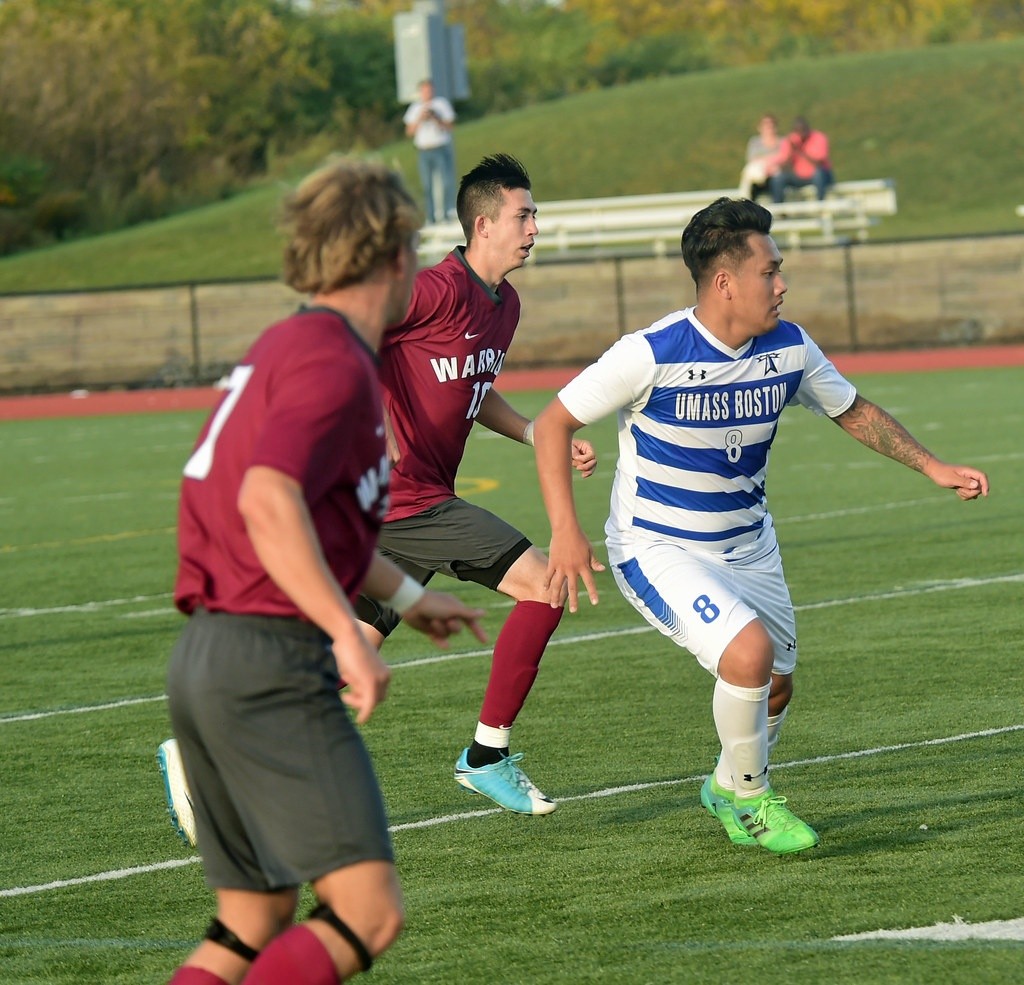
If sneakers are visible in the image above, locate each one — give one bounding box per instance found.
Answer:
[155,739,197,849]
[732,792,819,853]
[700,756,758,845]
[454,747,557,818]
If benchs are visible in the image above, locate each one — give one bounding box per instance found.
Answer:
[416,175,899,277]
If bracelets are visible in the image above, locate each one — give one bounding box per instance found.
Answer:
[523,421,534,447]
[382,574,426,616]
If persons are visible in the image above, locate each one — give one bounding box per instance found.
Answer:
[738,112,832,219]
[401,83,457,224]
[533,196,989,853]
[166,149,420,985]
[158,152,598,853]
